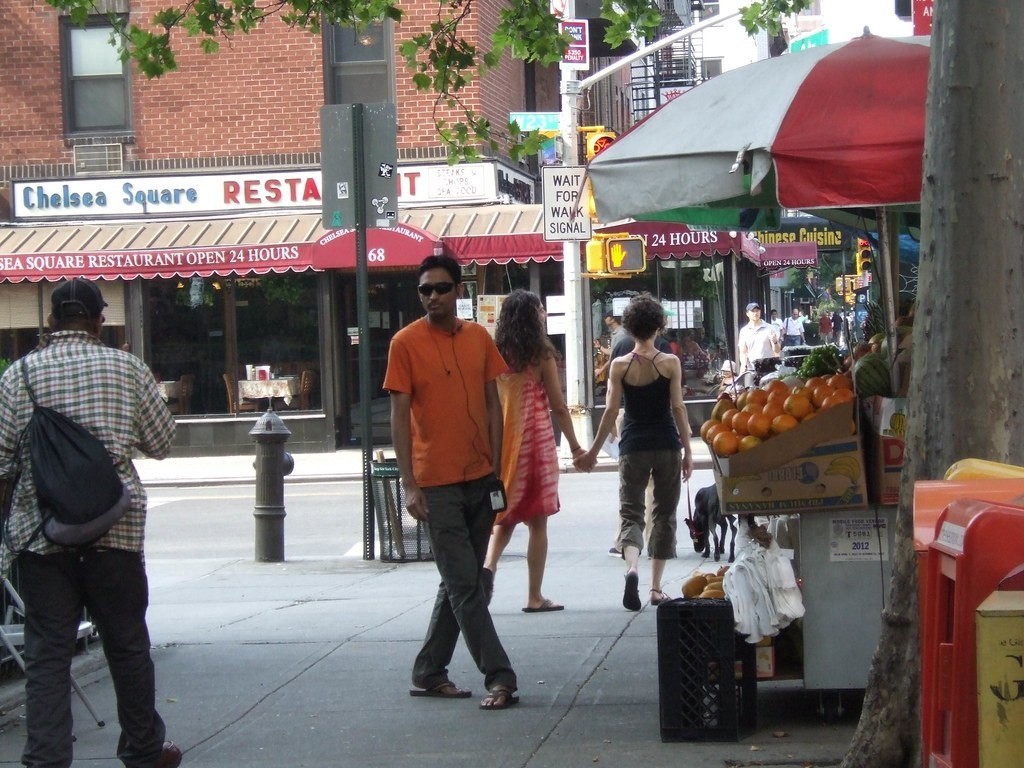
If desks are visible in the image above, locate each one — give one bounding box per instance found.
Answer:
[156,381,180,403]
[238,376,300,412]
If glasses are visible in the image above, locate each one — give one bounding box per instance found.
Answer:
[418,282,456,296]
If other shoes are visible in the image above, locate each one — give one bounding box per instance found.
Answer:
[125,741,184,768]
[649,589,672,605]
[608,547,623,558]
[623,573,642,611]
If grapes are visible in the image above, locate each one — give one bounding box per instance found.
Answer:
[753,345,843,388]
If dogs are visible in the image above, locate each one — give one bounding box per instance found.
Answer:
[684,487,736,563]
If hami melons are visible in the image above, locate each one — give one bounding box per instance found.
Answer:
[682,565,729,599]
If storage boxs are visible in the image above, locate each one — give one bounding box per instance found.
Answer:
[863,396,907,502]
[708,392,868,516]
[707,638,773,682]
[657,597,757,741]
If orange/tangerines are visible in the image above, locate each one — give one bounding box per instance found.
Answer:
[699,373,858,456]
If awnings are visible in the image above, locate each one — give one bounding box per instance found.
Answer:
[594,217,818,271]
[0,203,565,283]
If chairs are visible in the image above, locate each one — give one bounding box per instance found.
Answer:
[166,375,195,415]
[223,371,258,412]
[0,578,105,741]
[275,371,316,411]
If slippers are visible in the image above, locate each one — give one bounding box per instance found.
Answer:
[410,681,472,698]
[480,686,520,709]
[521,599,566,612]
[483,567,493,606]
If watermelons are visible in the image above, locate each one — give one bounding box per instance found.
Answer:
[852,354,892,399]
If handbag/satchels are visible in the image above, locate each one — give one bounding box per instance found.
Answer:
[780,340,785,350]
[27,406,132,546]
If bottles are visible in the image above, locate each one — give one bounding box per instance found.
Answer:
[251,368,256,380]
[271,371,274,379]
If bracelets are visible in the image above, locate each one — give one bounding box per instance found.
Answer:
[571,445,581,453]
[599,345,601,350]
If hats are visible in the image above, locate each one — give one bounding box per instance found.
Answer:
[746,302,761,311]
[721,360,737,373]
[51,277,110,316]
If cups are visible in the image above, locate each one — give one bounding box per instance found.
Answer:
[245,365,253,380]
[259,370,265,380]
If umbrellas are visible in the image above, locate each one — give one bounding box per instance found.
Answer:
[586,26,931,396]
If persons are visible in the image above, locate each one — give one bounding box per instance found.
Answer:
[482,289,595,613]
[0,279,182,768]
[382,256,519,710]
[572,295,693,610]
[718,303,854,399]
[589,309,673,560]
